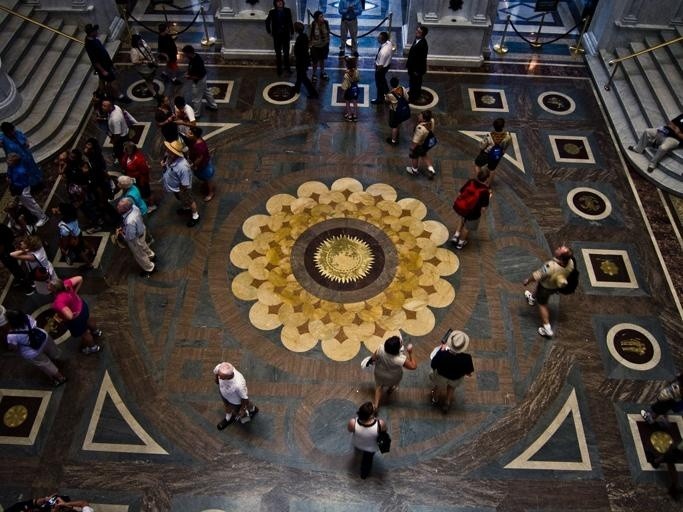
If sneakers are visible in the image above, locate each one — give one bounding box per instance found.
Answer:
[92,329,103,337]
[343,113,353,122]
[426,166,436,179]
[312,74,317,80]
[456,240,469,249]
[524,289,537,307]
[352,113,359,122]
[80,344,102,354]
[538,325,554,337]
[406,166,420,176]
[450,235,458,243]
[35,215,50,227]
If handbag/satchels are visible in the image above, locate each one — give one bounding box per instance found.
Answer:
[26,254,50,282]
[377,419,391,453]
[57,224,78,249]
[426,132,437,149]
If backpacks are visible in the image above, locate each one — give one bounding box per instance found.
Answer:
[12,322,47,349]
[453,181,485,218]
[561,265,580,296]
[392,90,412,122]
[490,145,504,163]
[349,82,359,99]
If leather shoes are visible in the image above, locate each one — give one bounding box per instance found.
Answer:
[187,212,200,227]
[144,265,157,279]
[647,165,655,172]
[203,192,215,202]
[176,207,191,214]
[371,98,381,104]
[217,415,235,430]
[627,145,638,153]
[248,405,259,420]
[149,254,157,261]
[386,137,396,146]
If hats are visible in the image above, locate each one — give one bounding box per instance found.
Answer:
[448,329,470,354]
[163,139,186,158]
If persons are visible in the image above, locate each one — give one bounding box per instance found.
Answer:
[522,247,580,337]
[263,1,397,124]
[450,170,502,251]
[347,328,475,480]
[4,493,94,512]
[407,26,429,101]
[472,118,510,188]
[385,77,411,146]
[628,113,683,171]
[212,361,259,429]
[640,375,683,501]
[405,110,439,179]
